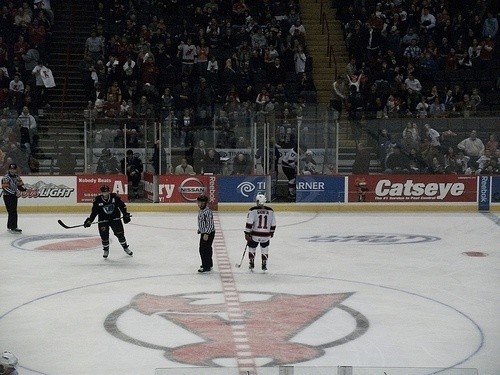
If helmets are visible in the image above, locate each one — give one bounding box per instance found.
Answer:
[198,195,208,202]
[256,194,266,205]
[305,151,313,156]
[9,164,17,169]
[0,352,17,368]
[100,185,109,192]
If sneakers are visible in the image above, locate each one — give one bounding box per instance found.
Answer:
[288,191,296,199]
[124,248,132,257]
[198,268,211,272]
[262,264,267,272]
[11,228,22,234]
[200,265,213,270]
[249,261,254,271]
[103,250,109,259]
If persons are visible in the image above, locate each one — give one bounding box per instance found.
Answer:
[244,195,276,270]
[197,195,215,272]
[329,0,500,179]
[86,0,318,196]
[0,0,56,175]
[84,185,133,258]
[1,164,24,232]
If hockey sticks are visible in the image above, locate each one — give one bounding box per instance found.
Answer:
[58,215,132,229]
[235,240,249,268]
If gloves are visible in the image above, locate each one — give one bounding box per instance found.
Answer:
[245,232,253,241]
[84,218,92,228]
[271,232,274,237]
[123,213,131,224]
